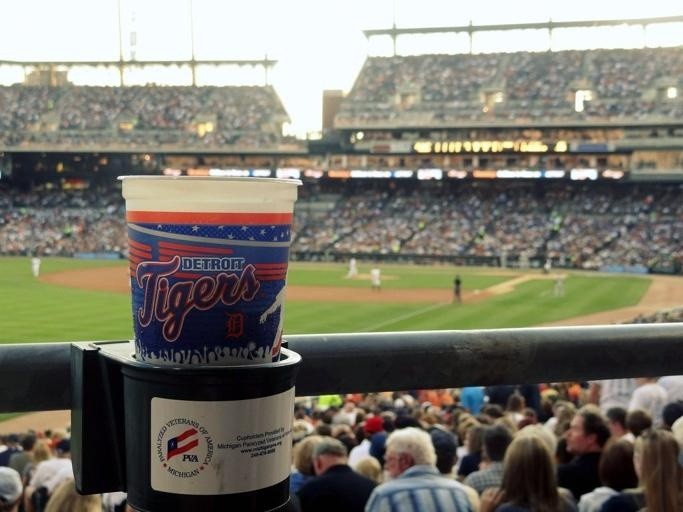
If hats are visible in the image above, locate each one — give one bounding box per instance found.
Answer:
[0,466,25,505]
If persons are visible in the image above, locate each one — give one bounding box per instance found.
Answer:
[1,424,128,511]
[553,274,565,297]
[370,267,380,291]
[347,256,358,280]
[452,274,463,304]
[292,376,683,511]
[32,256,41,278]
[1,45,683,274]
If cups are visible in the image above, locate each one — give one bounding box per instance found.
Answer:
[115,175,304,367]
